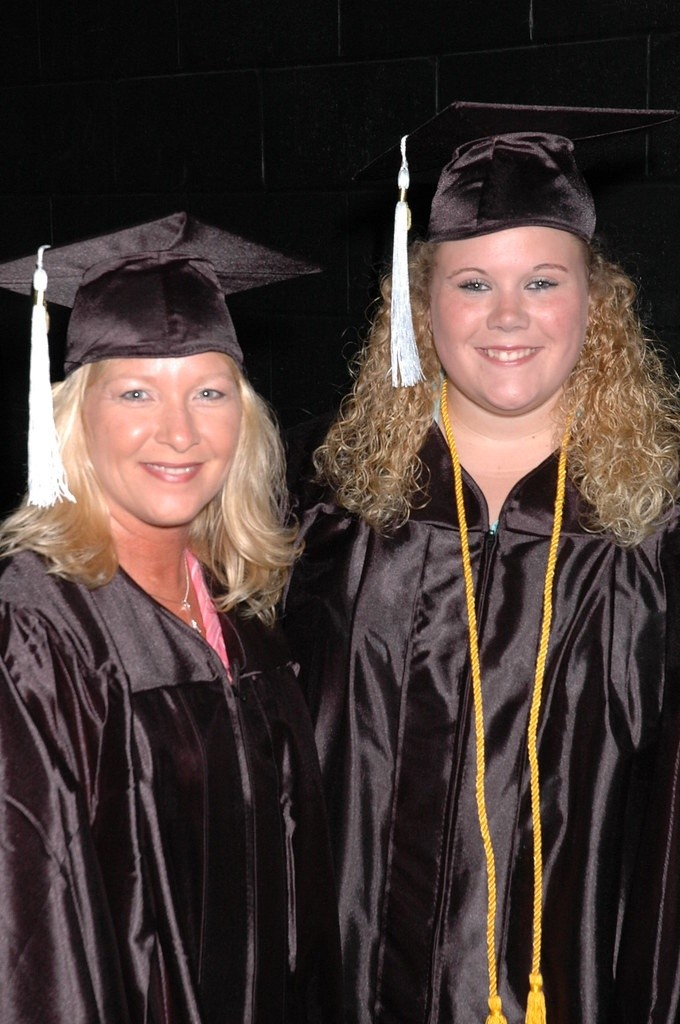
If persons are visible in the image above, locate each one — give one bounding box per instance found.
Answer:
[303,100,680,1024]
[0,211,359,1024]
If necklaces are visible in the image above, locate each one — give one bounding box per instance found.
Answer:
[140,556,198,632]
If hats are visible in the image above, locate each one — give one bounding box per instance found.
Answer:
[0,211,324,504]
[352,101,680,391]
